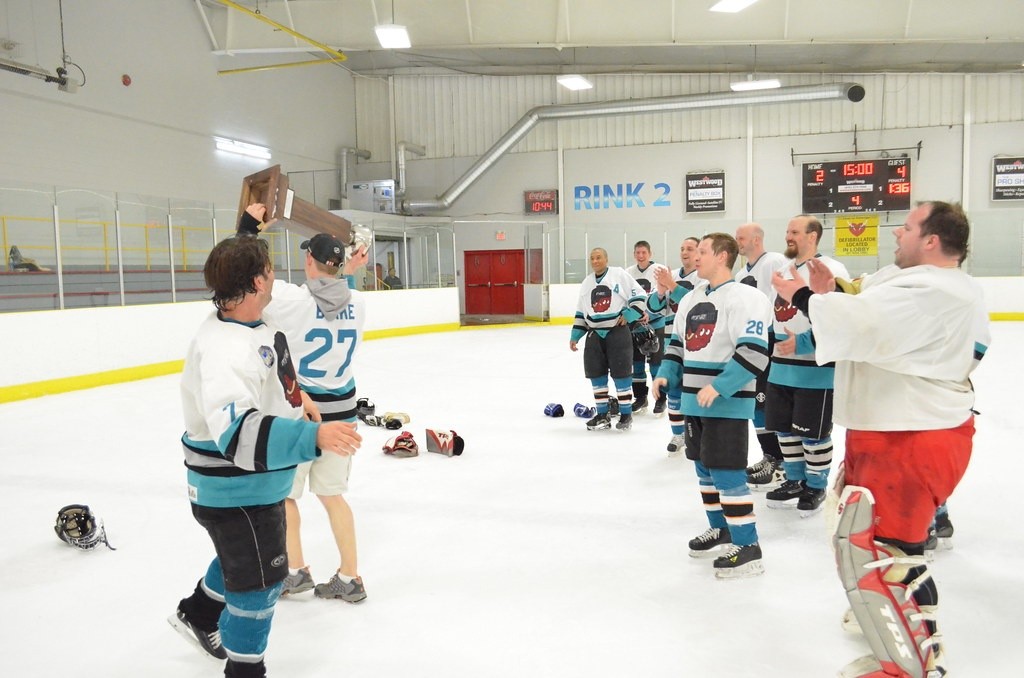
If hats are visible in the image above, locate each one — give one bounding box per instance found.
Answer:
[300,233,346,267]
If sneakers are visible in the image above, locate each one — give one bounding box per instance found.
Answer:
[279,565,316,595]
[585,413,612,431]
[713,540,765,577]
[923,511,954,562]
[839,605,950,678]
[631,394,649,414]
[745,455,787,491]
[795,482,828,518]
[666,432,686,457]
[168,580,227,662]
[615,413,633,432]
[688,526,733,557]
[314,568,367,602]
[652,391,667,417]
[765,480,805,510]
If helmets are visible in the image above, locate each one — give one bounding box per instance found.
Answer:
[608,395,619,414]
[53,503,116,553]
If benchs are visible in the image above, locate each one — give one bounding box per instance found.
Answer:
[0,265,306,312]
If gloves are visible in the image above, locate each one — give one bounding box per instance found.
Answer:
[543,402,564,417]
[573,403,596,418]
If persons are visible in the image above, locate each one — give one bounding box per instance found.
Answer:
[235,203,369,604]
[568,215,992,561]
[651,233,769,579]
[383,268,403,289]
[168,232,362,678]
[771,201,975,678]
[10,246,39,271]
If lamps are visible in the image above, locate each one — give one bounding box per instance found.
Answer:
[214,136,272,160]
[556,48,593,90]
[730,45,781,91]
[375,0,412,49]
